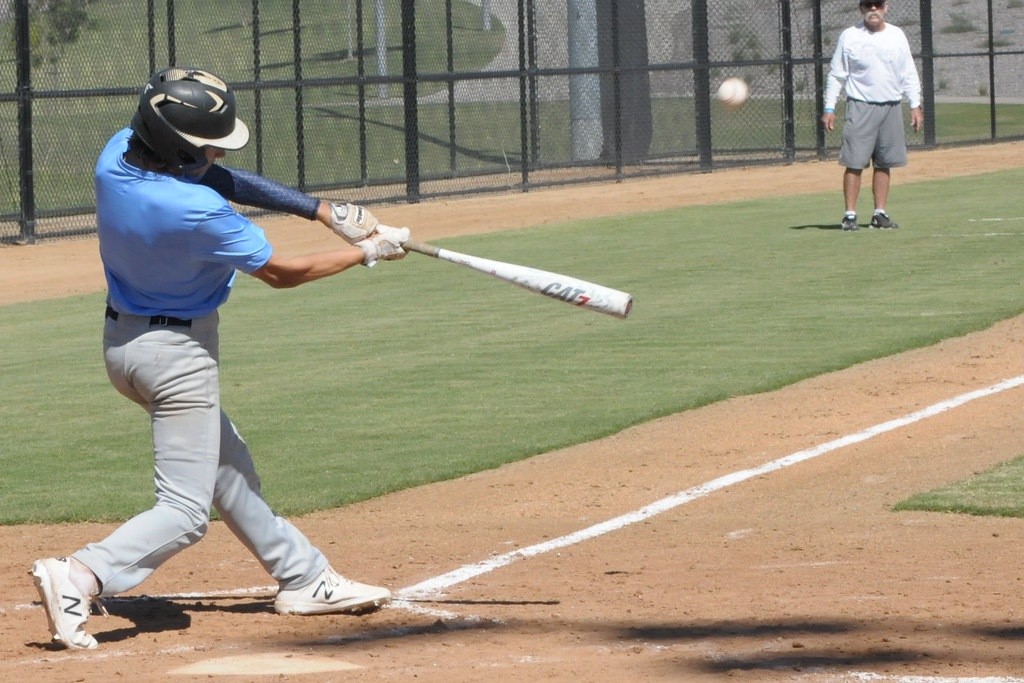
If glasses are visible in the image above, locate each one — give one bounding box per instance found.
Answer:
[860,0,883,9]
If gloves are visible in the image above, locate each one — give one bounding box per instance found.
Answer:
[329,200,378,243]
[357,223,411,267]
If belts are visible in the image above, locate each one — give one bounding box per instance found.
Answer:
[107,306,192,327]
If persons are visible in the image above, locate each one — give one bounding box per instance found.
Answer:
[821,0,923,231]
[26,65,411,650]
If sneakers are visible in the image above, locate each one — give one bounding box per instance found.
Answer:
[869,213,898,229]
[842,215,858,232]
[28,556,98,649]
[274,564,391,615]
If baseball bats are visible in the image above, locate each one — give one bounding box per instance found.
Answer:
[388,234,635,320]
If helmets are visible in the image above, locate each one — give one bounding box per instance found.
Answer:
[132,67,249,170]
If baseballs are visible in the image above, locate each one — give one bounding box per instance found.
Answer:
[717,78,748,105]
